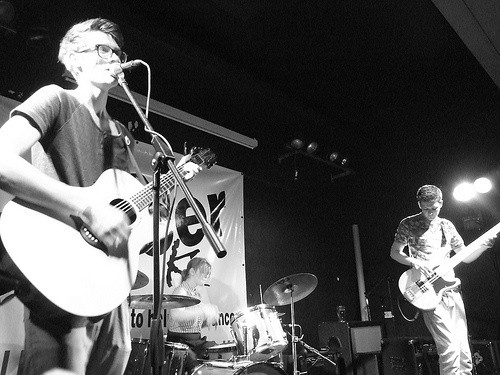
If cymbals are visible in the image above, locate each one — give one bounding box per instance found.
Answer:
[263,273,318,306]
[128,294,202,310]
[130,269,149,291]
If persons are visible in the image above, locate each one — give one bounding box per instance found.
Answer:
[391,185,496,375]
[0,16,201,374]
[166,257,219,375]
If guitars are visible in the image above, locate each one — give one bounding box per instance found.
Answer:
[0,147,219,329]
[396,222,500,311]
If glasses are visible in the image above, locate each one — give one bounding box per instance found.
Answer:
[77,45,128,63]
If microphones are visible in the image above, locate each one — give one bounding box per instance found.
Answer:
[109,57,140,77]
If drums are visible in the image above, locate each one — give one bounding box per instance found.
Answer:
[123,338,189,375]
[230,304,289,362]
[191,359,288,375]
